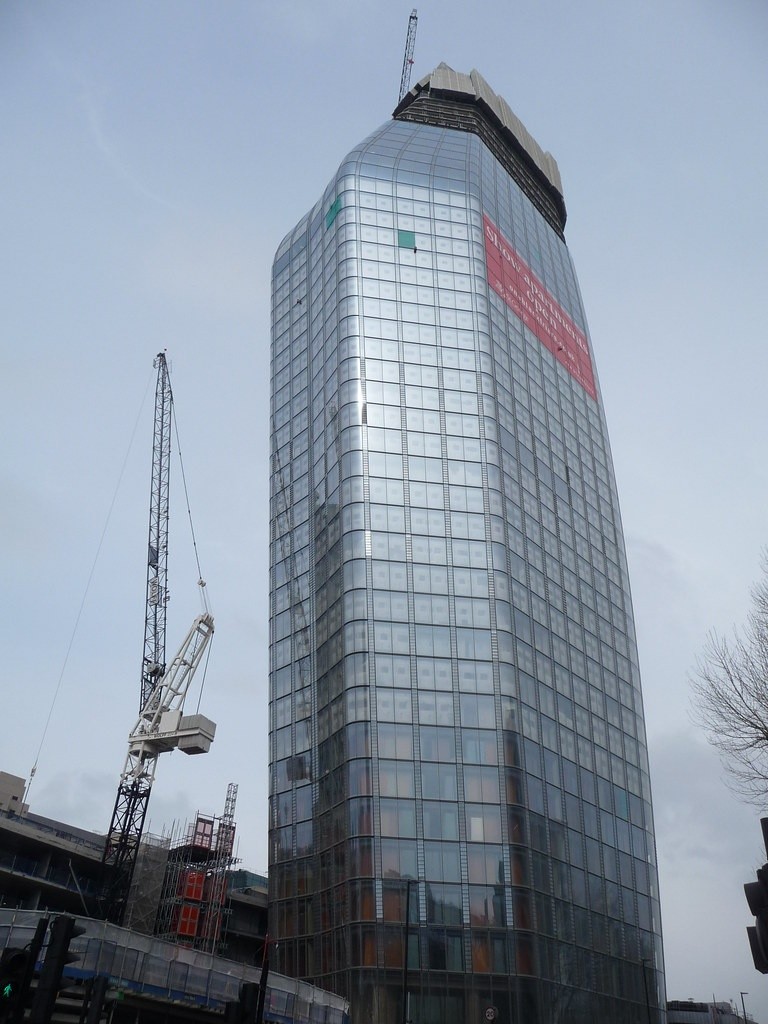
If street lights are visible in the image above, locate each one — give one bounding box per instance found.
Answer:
[642,958,651,1024]
[740,992,748,1024]
[401,881,420,1022]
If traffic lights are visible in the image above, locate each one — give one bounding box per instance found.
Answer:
[44,918,86,995]
[741,863,768,973]
[225,983,259,1024]
[0,948,30,1024]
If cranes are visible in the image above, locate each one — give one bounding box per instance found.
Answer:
[89,352,220,976]
